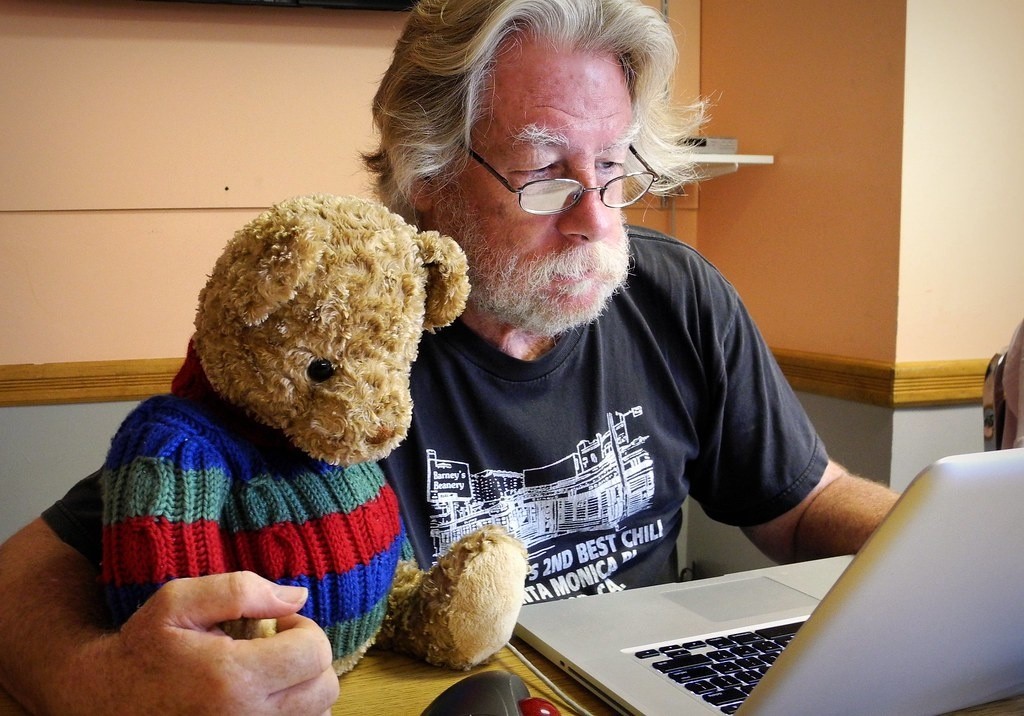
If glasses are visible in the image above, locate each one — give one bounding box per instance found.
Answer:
[460,143,660,216]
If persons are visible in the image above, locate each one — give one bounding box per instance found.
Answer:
[0,0,911,714]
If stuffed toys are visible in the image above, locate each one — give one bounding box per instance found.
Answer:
[98,192,529,682]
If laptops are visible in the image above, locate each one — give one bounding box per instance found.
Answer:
[513,449,1024,716]
